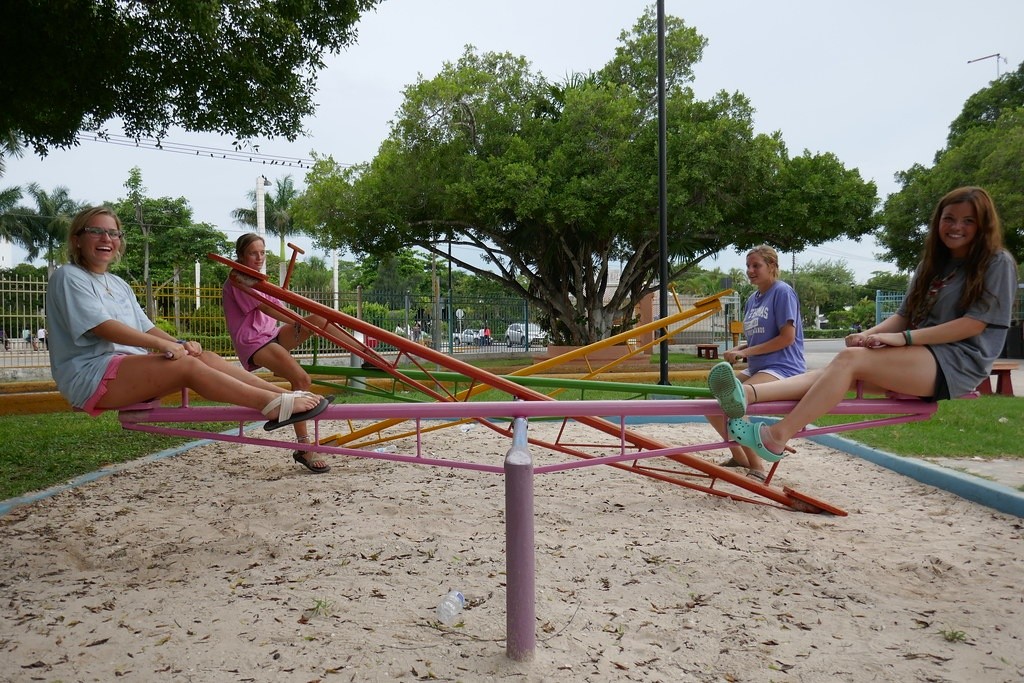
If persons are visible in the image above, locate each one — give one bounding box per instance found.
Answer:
[867,323,871,330]
[224,233,399,472]
[703,245,806,483]
[46,208,335,430]
[484,326,490,345]
[38,325,49,350]
[413,320,420,343]
[0,329,9,350]
[23,326,38,351]
[708,186,1018,461]
[857,323,862,333]
[480,326,484,346]
[395,323,404,335]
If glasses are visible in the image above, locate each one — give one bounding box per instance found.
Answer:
[76,226,123,241]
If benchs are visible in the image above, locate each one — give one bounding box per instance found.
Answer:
[697,344,719,359]
[977,362,1018,396]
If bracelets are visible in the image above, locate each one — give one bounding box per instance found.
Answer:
[177,339,186,344]
[902,330,908,345]
[907,329,912,346]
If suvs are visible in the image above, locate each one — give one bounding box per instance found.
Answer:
[504,323,549,347]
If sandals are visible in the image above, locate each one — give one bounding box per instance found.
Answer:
[362,355,399,370]
[260,392,330,431]
[292,390,335,404]
[293,450,331,473]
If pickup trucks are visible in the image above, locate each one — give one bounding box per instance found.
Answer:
[453,328,493,346]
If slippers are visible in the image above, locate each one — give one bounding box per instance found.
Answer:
[746,470,770,484]
[727,417,784,463]
[708,360,746,419]
[720,458,744,467]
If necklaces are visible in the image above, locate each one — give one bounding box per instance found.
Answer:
[89,269,114,298]
